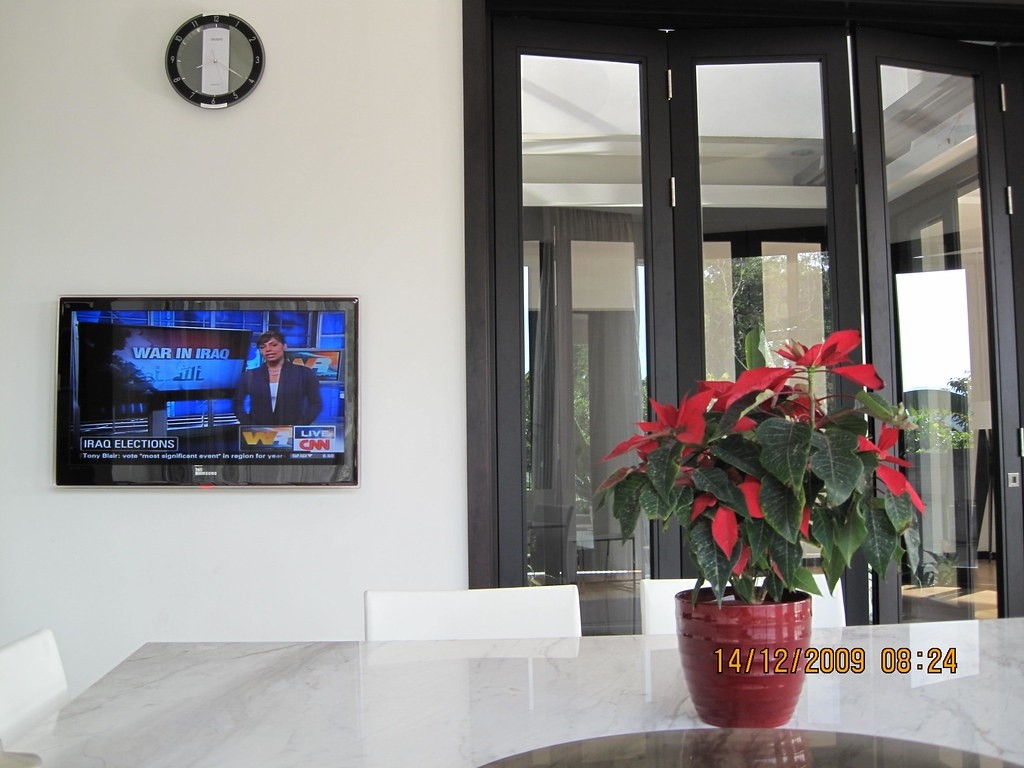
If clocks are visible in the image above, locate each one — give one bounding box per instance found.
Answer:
[165,13,266,110]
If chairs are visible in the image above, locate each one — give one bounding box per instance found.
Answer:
[528,504,574,586]
[0,624,70,726]
[358,580,584,643]
[637,572,848,636]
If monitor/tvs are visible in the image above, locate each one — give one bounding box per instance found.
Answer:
[56,295,361,491]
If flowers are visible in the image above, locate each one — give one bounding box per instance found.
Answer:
[584,328,928,611]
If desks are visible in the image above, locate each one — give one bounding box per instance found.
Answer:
[526,519,568,529]
[0,614,1024,768]
[568,532,636,591]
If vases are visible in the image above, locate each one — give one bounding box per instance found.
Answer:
[673,582,816,727]
[680,727,814,768]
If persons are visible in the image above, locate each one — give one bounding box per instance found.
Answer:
[231,331,323,425]
